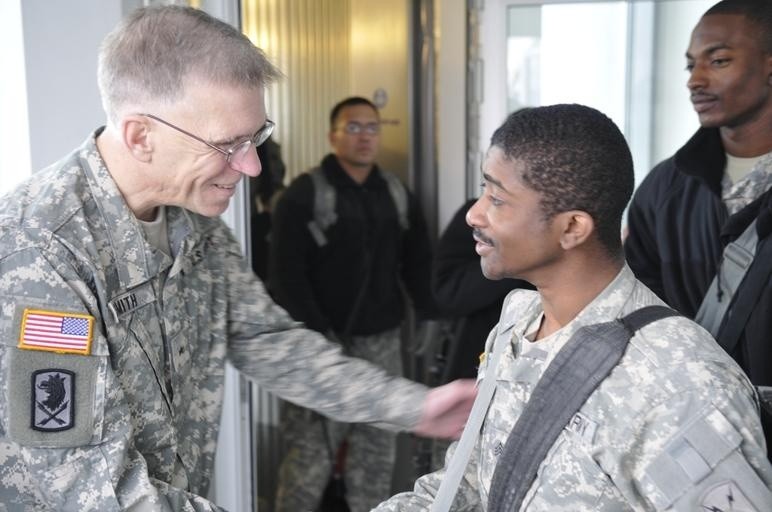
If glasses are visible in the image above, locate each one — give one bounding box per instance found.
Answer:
[139,111,279,168]
[334,122,380,136]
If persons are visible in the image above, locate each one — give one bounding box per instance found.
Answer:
[429,198,537,472]
[365,104,772,512]
[0,5,478,512]
[266,98,442,512]
[624,0,772,463]
